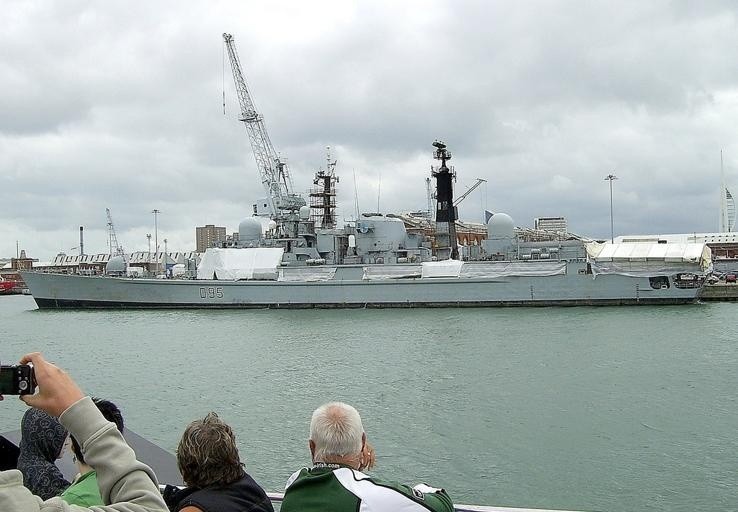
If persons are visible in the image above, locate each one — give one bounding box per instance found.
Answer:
[16,406,73,500]
[162,411,276,511]
[0,352,170,512]
[275,401,455,511]
[55,396,125,507]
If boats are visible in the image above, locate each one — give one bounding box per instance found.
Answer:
[0,272,27,295]
[146,233,168,256]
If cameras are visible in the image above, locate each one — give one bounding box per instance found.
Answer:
[0,364,35,395]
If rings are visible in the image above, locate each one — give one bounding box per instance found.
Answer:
[367,452,371,456]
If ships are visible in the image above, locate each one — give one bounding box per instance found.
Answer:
[17,29,719,311]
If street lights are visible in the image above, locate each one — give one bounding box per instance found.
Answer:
[151,209,161,262]
[604,173,618,244]
